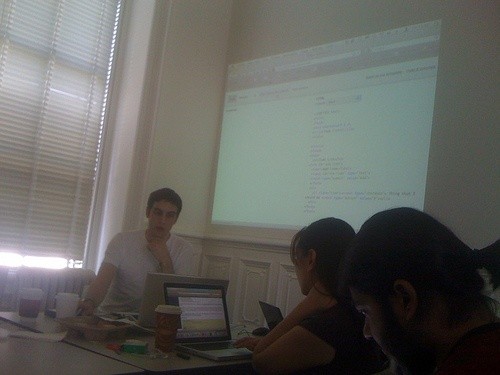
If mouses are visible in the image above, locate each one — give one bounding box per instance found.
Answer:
[252,327,268,334]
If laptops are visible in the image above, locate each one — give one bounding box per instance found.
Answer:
[164,282,253,361]
[258,300,287,331]
[111,271,229,328]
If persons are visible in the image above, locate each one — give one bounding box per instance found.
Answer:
[79,188,193,316]
[338,208,499,375]
[233,217,391,375]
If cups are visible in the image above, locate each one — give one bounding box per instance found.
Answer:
[154,305,182,353]
[54,292,85,319]
[18,288,43,320]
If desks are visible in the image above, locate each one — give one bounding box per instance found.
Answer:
[0,311,262,375]
